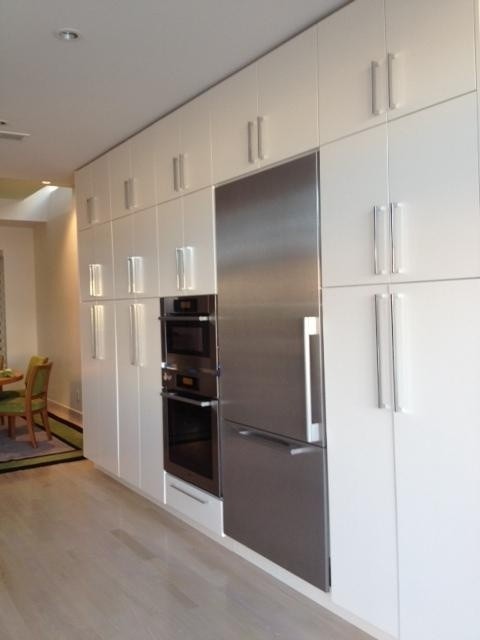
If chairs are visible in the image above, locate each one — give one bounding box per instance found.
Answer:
[1,350,52,448]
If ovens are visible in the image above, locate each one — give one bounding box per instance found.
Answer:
[158,296,218,498]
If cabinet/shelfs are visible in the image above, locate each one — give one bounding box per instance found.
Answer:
[104,119,157,219]
[318,276,478,639]
[319,0,477,145]
[319,90,479,289]
[69,153,112,232]
[75,303,120,483]
[74,219,115,301]
[154,89,213,204]
[212,22,320,184]
[115,299,168,504]
[154,185,217,296]
[110,204,160,298]
